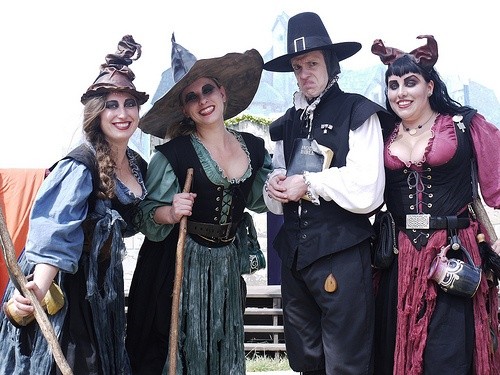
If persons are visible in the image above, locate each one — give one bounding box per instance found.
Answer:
[0,72,148,375]
[261,11,396,375]
[125,45,273,375]
[383,53,500,375]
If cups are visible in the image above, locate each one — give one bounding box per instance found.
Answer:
[427,243,482,299]
[3,273,65,329]
[241,239,266,275]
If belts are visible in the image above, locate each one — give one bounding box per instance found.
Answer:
[187,218,241,239]
[395,207,471,233]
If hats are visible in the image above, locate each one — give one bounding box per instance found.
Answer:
[80,34,150,105]
[137,32,263,140]
[262,10,361,72]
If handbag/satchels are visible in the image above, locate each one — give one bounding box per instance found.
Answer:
[369,209,395,267]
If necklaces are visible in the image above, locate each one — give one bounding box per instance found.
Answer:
[402,112,434,135]
